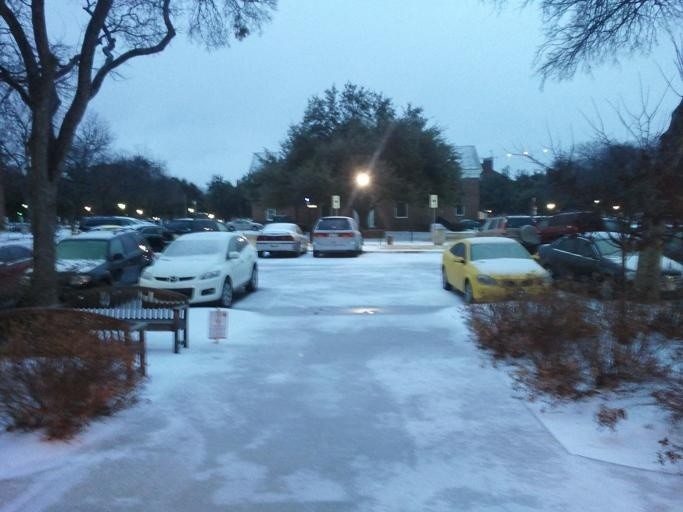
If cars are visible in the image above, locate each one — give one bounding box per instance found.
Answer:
[441,233,554,308]
[652,224,683,264]
[539,231,683,299]
[7,215,230,251]
[257,223,307,255]
[226,217,262,232]
[137,229,259,311]
[0,232,35,306]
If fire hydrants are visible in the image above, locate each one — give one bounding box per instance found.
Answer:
[387,234,394,246]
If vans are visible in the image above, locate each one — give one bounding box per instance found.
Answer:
[311,214,364,258]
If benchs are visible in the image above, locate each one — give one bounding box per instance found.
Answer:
[56,282,191,354]
[0,306,149,388]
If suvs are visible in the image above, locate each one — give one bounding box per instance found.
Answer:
[459,211,649,252]
[57,222,153,309]
